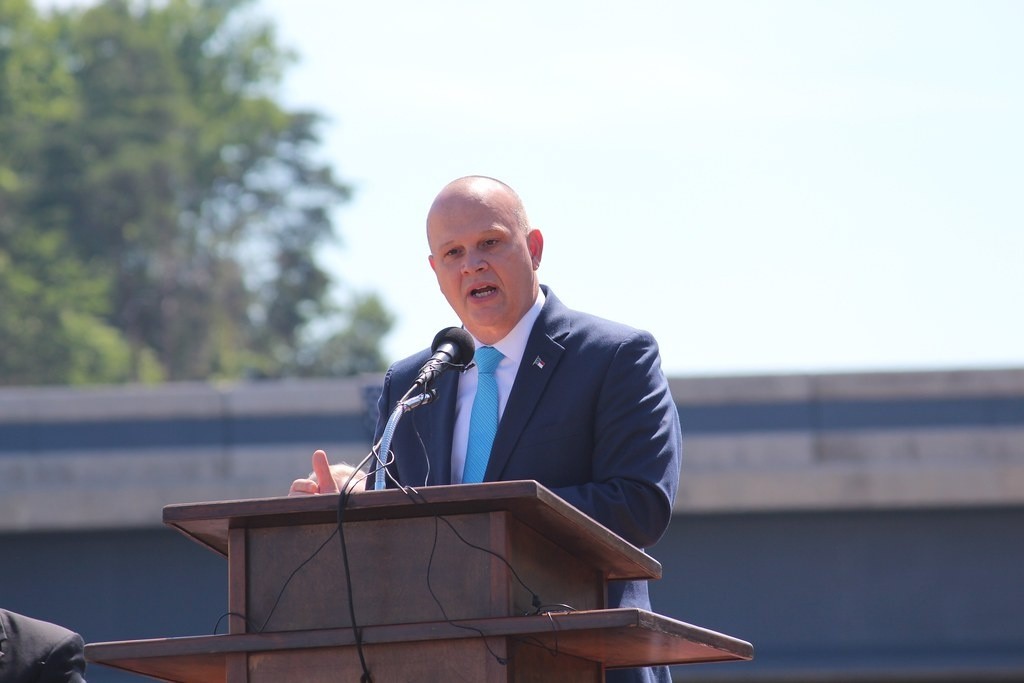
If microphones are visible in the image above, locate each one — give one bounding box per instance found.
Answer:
[415,327,475,386]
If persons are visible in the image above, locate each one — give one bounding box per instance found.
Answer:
[0,609,87,683]
[287,174,682,683]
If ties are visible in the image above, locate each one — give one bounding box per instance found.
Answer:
[461,347,506,484]
